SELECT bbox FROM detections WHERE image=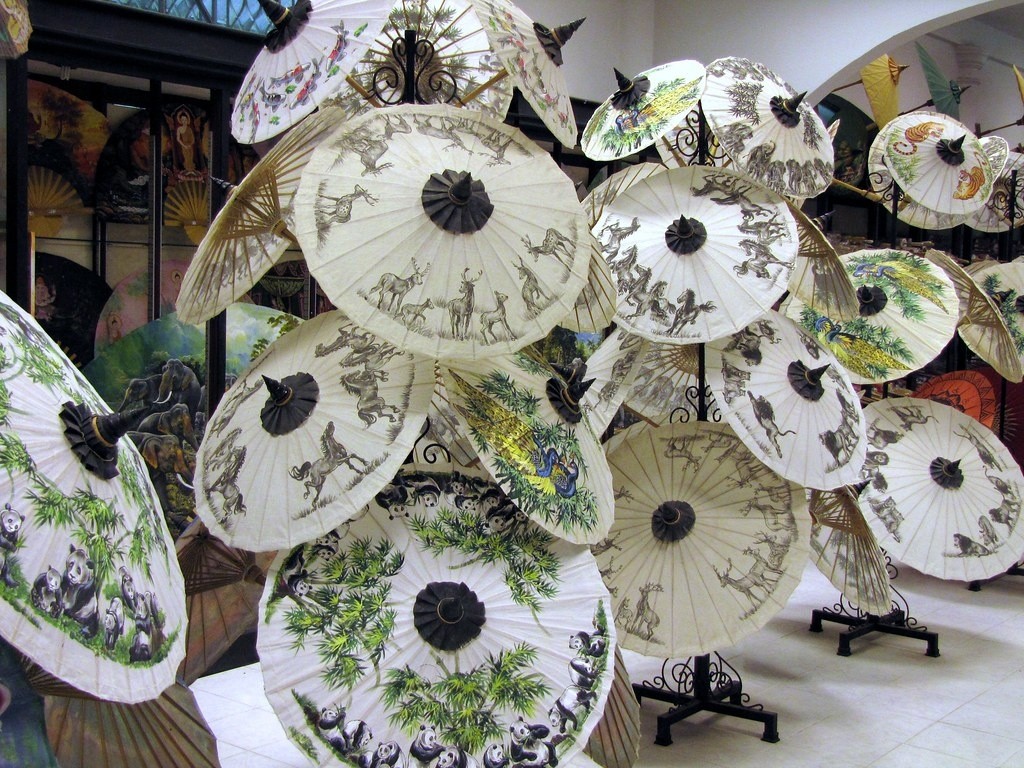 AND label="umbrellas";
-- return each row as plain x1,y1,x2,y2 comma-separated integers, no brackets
294,104,591,358
1,1,1021,768
